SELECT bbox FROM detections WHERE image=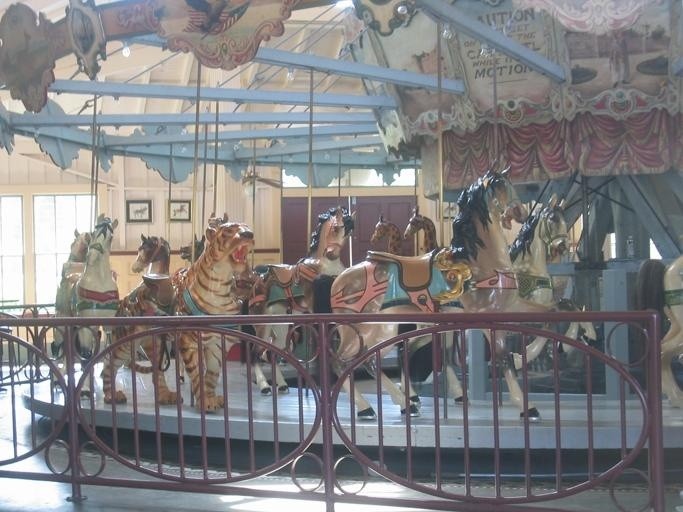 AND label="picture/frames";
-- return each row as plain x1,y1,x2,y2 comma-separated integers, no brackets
168,200,191,222
126,199,153,222
